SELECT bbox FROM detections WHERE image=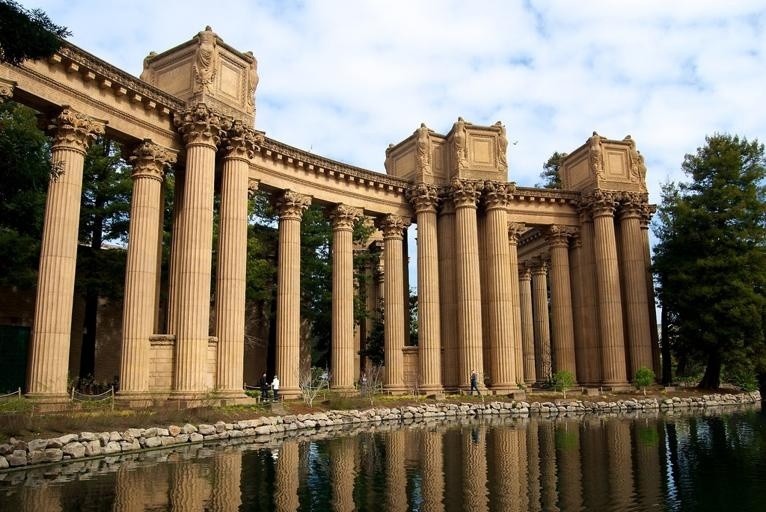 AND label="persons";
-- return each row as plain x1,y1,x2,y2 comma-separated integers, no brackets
260,372,270,402
271,376,280,400
469,370,481,395
